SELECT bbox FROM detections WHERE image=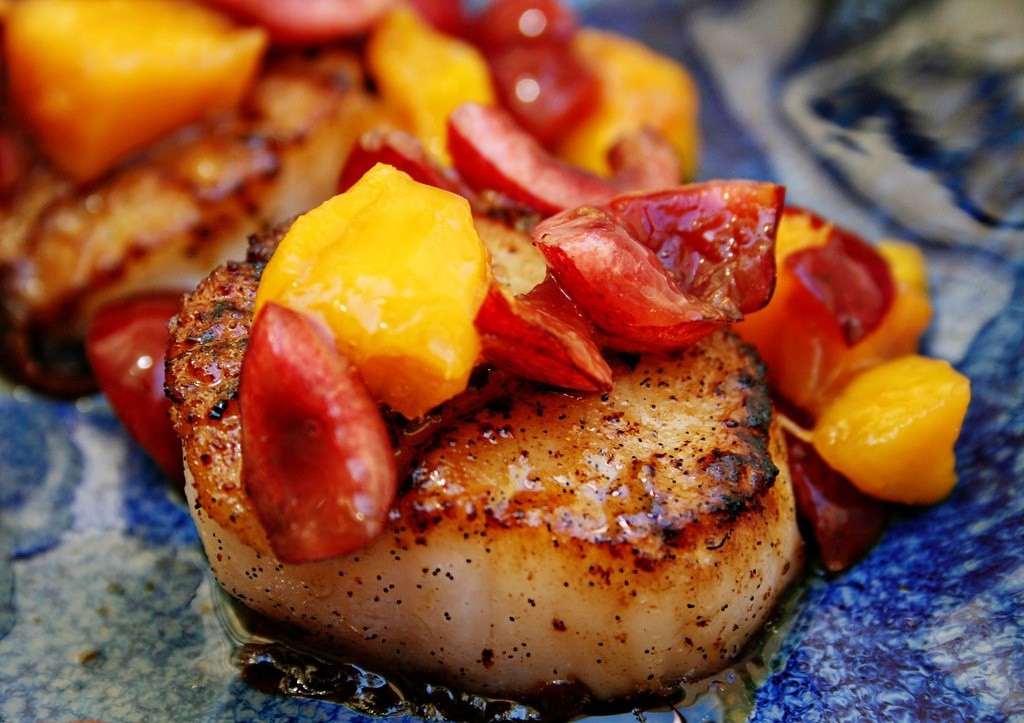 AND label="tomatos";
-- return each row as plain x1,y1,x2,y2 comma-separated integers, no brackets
83,0,785,564
